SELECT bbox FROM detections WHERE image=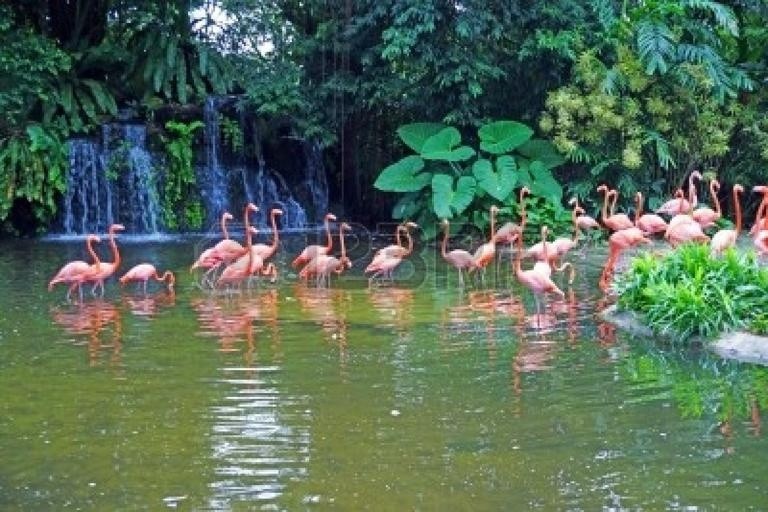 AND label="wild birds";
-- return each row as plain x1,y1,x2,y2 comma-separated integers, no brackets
361,219,419,282
118,261,176,298
489,186,533,254
533,226,554,281
46,234,102,306
293,211,353,283
527,242,575,288
67,223,126,298
553,206,585,251
191,201,286,290
369,222,407,282
566,169,768,267
510,247,568,325
470,205,501,281
437,218,483,289
